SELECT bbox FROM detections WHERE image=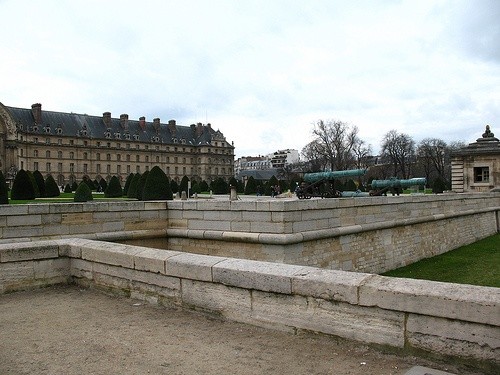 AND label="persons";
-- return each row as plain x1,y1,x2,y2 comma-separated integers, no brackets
61,184,64,191
254,181,344,201
